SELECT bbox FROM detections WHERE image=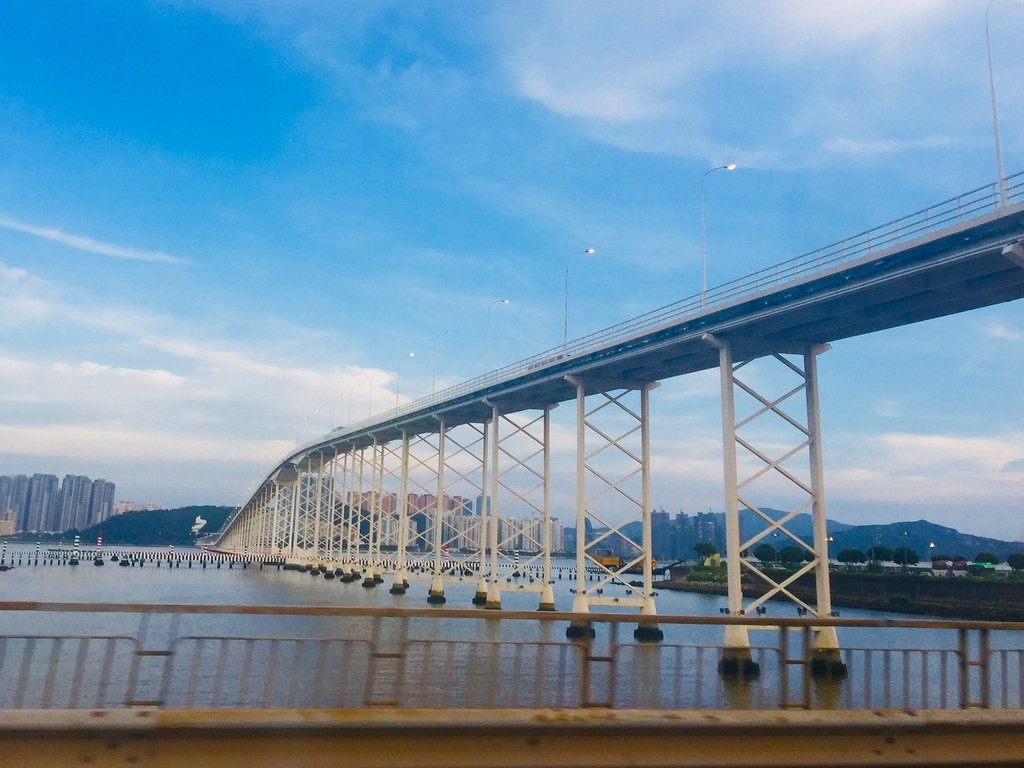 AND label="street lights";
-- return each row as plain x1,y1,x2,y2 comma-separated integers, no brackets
432,331,449,405
484,298,510,392
699,164,739,314
771,533,780,567
902,531,908,571
396,352,415,418
563,248,595,360
